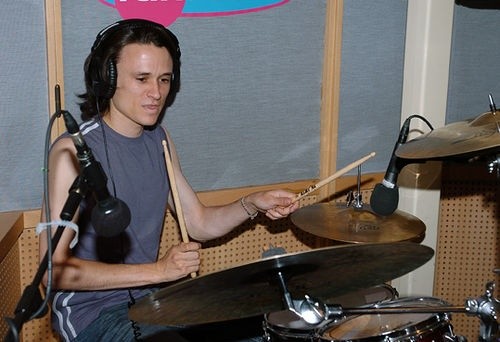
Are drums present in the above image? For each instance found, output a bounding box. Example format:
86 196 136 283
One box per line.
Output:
315 295 457 342
263 282 399 342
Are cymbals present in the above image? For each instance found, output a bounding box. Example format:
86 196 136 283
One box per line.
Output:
396 109 500 161
292 202 426 243
127 241 435 325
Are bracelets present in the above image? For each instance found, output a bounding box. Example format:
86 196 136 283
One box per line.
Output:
241 197 258 220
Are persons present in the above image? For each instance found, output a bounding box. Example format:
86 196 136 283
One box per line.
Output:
37 20 300 342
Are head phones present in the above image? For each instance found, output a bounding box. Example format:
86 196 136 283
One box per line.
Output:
84 18 183 103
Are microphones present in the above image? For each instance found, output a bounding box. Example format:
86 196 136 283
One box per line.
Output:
370 118 410 216
63 112 131 238
